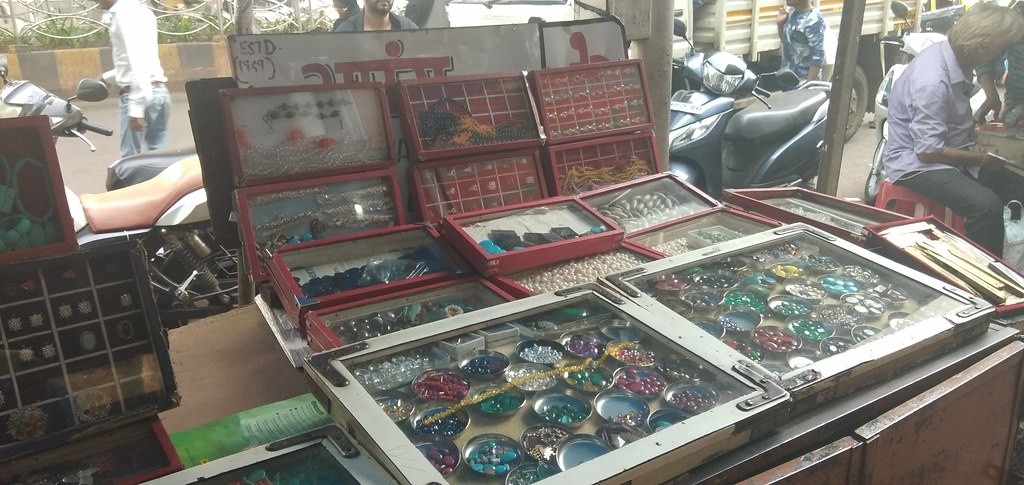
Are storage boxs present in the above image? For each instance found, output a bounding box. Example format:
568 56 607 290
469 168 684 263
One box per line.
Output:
138 422 401 485
215 57 1023 485
0 114 184 485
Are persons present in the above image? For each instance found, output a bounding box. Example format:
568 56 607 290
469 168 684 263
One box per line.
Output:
883 0 1024 260
332 0 419 31
96 0 172 158
776 0 825 83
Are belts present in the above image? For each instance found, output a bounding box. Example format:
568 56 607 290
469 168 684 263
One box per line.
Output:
119 82 164 96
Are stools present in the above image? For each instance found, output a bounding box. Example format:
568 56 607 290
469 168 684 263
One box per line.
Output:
875 179 967 232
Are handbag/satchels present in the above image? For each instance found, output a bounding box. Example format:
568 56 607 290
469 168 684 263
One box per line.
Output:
1002 200 1024 274
817 27 837 82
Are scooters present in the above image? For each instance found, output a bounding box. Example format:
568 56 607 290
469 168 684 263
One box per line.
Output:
0 54 239 328
864 0 989 209
668 8 834 201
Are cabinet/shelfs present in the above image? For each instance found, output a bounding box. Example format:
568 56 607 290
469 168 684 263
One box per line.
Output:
672 326 1023 484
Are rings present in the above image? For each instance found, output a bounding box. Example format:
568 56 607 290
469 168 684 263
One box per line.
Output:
422 157 536 214
0 263 134 362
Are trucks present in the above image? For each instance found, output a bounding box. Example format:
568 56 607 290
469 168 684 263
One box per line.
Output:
439 1 922 146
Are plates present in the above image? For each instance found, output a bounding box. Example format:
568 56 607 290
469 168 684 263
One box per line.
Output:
370 242 949 485
